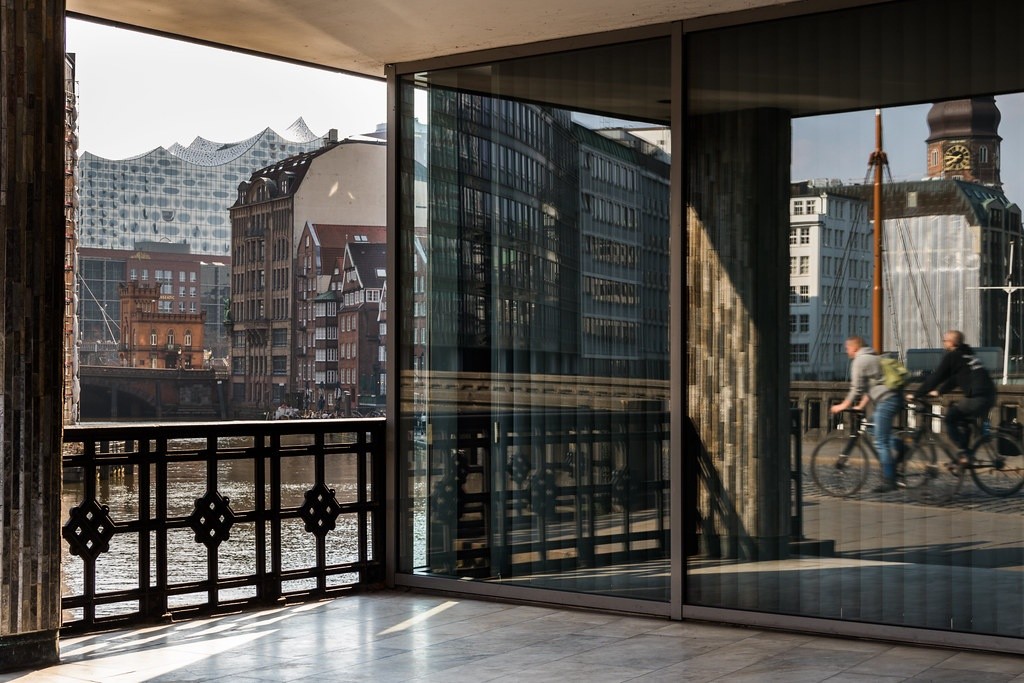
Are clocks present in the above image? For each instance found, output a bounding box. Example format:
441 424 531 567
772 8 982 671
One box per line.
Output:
943 144 971 171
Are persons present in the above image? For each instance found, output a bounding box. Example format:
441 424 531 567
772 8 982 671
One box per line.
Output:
911 329 1000 460
827 335 916 495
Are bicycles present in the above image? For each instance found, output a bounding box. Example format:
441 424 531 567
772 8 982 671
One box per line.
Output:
809 406 966 507
888 391 1024 496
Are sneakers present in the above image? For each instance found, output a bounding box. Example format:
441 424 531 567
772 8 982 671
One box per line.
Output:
947 448 969 466
894 445 909 462
872 479 898 492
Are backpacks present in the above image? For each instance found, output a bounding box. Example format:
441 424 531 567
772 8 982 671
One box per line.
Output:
880 356 910 390
